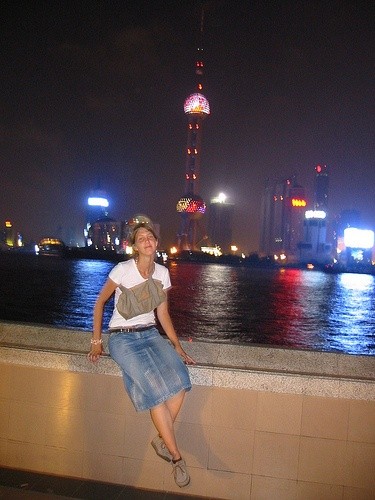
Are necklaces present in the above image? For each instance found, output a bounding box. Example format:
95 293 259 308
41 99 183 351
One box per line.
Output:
136 256 155 265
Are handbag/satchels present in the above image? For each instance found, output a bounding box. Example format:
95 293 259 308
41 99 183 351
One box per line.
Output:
116 278 166 320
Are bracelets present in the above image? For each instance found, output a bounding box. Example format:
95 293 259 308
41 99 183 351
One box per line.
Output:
91 338 103 345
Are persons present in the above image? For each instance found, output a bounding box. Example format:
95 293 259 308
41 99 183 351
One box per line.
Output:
89 223 195 487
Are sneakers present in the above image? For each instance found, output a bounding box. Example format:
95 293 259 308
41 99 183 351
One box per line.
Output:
150 436 171 462
172 458 190 488
